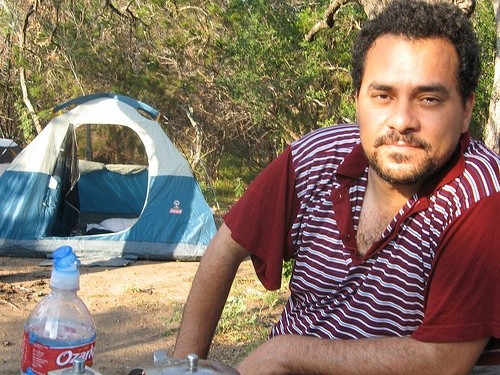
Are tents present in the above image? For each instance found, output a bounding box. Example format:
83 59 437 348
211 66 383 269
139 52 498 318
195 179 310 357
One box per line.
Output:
0 137 23 178
0 92 218 267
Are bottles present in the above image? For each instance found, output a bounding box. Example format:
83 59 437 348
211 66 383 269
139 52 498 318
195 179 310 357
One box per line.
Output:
19 246 97 375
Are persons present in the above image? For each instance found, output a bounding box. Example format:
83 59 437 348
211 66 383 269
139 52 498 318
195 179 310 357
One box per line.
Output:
168 0 500 375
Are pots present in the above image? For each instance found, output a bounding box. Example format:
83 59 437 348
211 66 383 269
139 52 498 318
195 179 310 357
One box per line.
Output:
144 349 240 375
